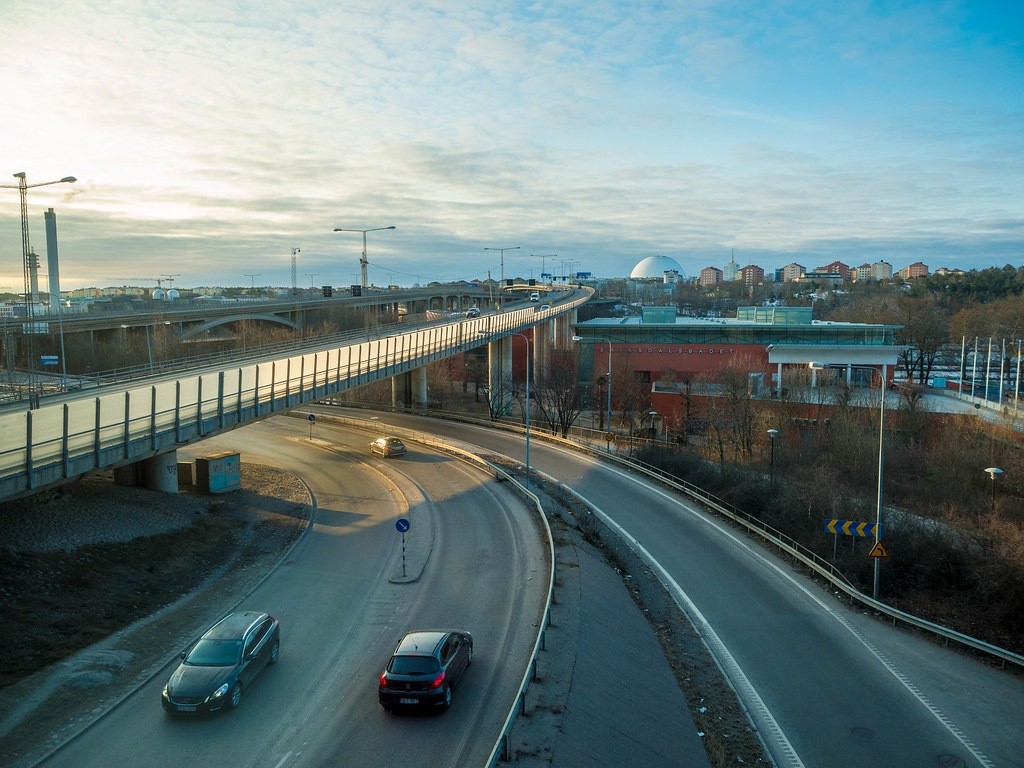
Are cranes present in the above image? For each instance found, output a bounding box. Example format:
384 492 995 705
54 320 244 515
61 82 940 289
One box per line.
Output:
106 277 174 288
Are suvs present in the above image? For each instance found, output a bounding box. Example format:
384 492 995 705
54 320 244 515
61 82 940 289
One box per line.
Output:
162 611 280 718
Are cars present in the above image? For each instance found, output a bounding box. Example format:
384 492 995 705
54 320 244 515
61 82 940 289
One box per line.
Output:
540 305 550 313
466 308 481 318
377 628 474 714
370 436 408 459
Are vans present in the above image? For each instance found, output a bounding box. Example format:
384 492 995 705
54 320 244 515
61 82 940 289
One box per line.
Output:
530 293 540 302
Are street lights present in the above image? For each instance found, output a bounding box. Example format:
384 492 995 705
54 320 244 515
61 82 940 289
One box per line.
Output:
0 171 77 314
333 226 397 290
349 273 362 285
386 273 397 285
245 274 262 287
984 468 1003 513
19 293 67 391
572 336 611 446
530 254 558 295
304 272 319 288
552 258 581 290
161 274 180 300
649 411 659 439
808 362 885 602
484 246 521 304
477 330 530 490
766 429 780 479
